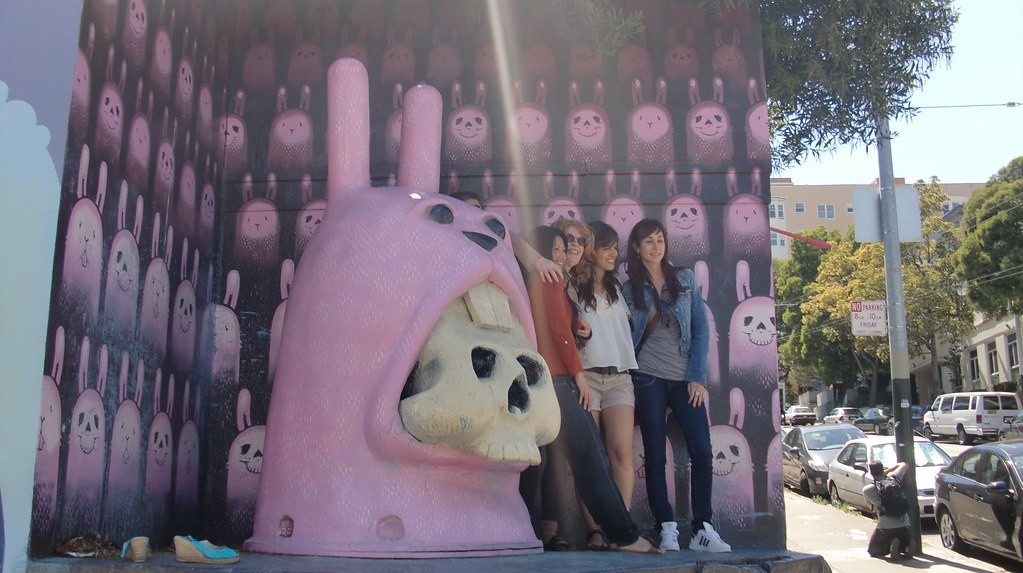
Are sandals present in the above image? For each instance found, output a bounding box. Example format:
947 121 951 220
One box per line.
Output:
544 533 568 551
587 531 608 550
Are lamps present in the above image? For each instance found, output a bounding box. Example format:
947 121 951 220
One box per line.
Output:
912 364 915 368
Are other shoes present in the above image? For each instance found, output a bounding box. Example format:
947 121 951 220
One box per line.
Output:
890 537 901 562
904 539 916 559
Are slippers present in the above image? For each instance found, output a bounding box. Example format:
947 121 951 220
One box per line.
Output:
616 537 665 554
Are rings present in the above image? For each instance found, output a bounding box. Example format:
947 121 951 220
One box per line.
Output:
695 391 699 395
540 272 543 274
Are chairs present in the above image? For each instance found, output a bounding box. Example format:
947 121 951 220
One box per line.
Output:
800 434 817 450
973 460 992 482
993 456 1021 488
826 431 846 446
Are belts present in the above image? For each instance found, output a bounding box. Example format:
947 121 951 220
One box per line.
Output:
588 366 624 375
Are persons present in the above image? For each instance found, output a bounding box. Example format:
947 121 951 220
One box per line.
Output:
863 460 916 561
459 191 666 553
621 218 730 553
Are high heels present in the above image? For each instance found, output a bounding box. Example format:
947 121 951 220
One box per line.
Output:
173 534 241 564
121 536 151 564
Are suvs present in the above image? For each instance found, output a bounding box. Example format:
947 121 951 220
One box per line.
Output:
924 390 1022 445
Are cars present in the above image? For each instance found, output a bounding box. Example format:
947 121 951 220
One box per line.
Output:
853 406 894 435
934 441 1023 561
782 425 875 499
781 404 818 426
822 406 863 426
887 405 931 435
827 436 968 523
998 410 1023 445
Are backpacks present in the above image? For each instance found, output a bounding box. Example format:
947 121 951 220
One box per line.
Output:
874 478 910 517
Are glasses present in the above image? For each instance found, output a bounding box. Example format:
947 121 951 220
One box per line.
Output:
566 233 587 246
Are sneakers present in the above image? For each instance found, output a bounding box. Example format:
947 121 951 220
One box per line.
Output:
658 520 680 551
688 522 731 553
